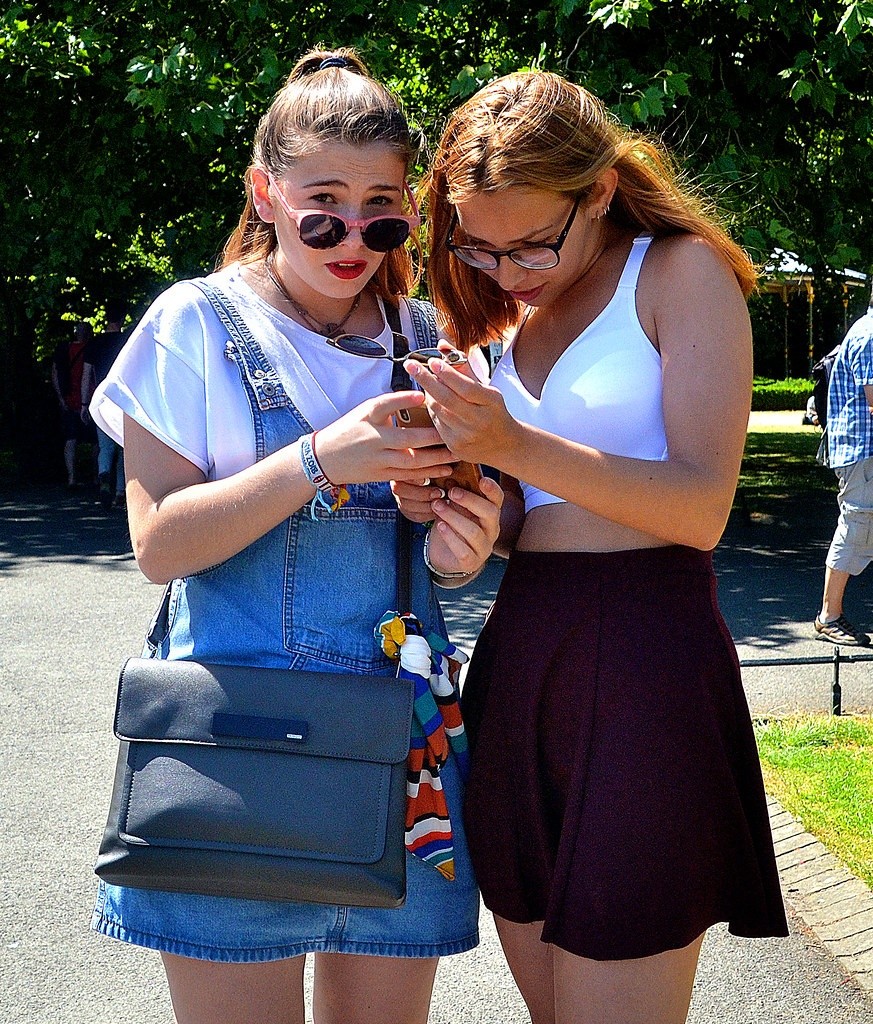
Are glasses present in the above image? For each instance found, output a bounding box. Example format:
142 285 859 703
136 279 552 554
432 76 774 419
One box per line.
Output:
325 334 468 368
267 171 421 255
444 190 581 271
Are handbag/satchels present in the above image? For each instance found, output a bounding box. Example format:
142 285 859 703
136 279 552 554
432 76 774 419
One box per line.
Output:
94 657 415 909
59 409 89 436
811 344 841 458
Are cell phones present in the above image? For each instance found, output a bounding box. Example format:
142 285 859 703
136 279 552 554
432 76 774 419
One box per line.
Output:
395 397 488 518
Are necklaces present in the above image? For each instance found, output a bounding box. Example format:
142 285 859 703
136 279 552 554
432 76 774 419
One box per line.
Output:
260 252 362 337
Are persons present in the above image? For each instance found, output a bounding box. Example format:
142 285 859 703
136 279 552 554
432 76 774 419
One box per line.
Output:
82 298 134 508
401 66 793 1024
813 283 873 647
50 321 96 491
82 45 508 1023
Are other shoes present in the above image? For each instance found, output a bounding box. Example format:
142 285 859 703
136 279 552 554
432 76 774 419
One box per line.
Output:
99 482 111 510
812 610 871 646
116 488 125 506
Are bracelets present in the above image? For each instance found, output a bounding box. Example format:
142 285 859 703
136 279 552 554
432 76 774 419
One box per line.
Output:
82 401 88 406
299 428 350 522
422 527 476 579
